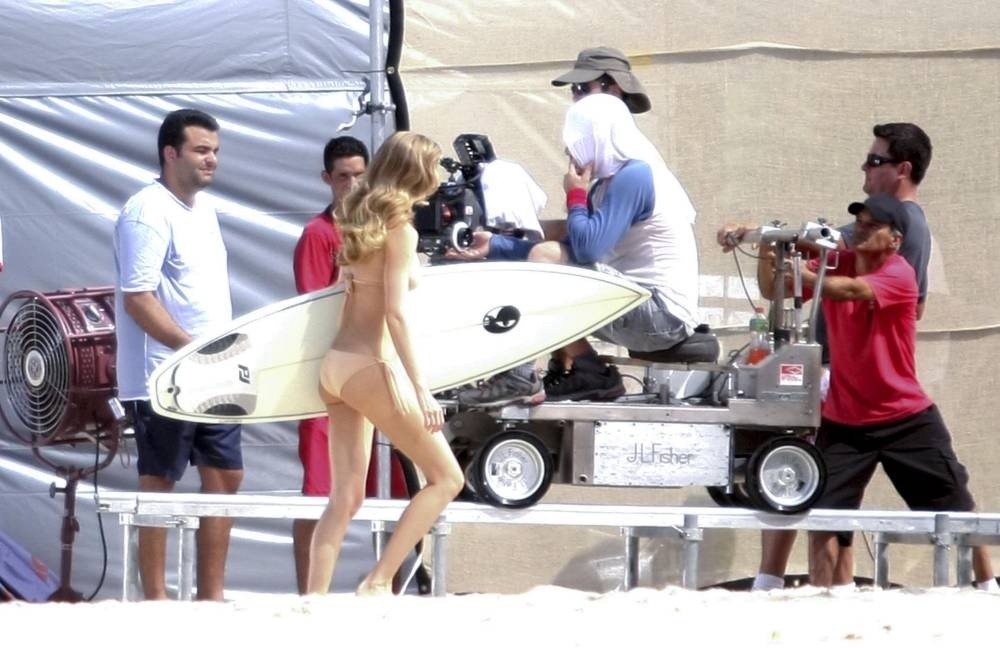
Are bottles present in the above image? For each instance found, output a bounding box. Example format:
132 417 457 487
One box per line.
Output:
749 305 772 366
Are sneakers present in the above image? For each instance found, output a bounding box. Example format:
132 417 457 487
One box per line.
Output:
541 349 626 403
457 369 545 408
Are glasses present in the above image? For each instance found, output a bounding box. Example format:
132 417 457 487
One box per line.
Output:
570 81 607 95
867 153 900 168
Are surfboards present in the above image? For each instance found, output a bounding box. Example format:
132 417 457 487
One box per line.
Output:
146 263 651 423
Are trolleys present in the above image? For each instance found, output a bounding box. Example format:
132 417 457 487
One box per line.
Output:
430 224 845 515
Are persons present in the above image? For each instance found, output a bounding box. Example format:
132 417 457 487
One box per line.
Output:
113 108 243 602
291 135 410 595
304 131 466 597
456 45 998 591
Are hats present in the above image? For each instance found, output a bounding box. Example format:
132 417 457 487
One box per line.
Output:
552 46 651 113
848 191 910 237
